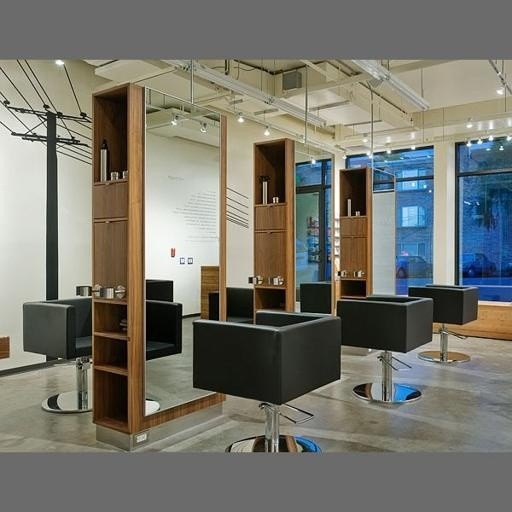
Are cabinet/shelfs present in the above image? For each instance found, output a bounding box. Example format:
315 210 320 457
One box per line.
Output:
92 83 142 435
338 167 371 303
252 140 294 318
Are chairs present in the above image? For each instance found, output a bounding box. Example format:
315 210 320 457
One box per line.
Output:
22 297 93 413
191 308 342 454
408 284 478 363
146 299 182 361
337 294 433 404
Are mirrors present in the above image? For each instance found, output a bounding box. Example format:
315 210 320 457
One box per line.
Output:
291 141 333 316
145 89 228 430
373 170 396 297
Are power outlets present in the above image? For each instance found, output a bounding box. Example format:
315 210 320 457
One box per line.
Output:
180 258 185 264
188 258 193 264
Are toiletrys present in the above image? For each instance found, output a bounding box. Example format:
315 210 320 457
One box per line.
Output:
110 172 119 180
123 171 129 179
272 197 278 204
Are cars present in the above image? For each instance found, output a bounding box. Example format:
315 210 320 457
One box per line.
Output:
395 255 432 279
462 252 497 278
